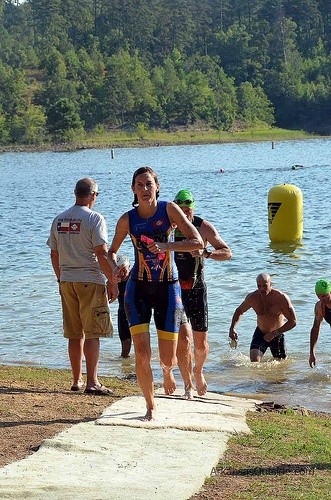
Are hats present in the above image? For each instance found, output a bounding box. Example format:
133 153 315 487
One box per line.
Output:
174 189 194 207
315 279 331 294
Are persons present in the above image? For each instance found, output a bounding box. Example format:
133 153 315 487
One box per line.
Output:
46 178 120 394
106 254 131 358
107 167 205 421
309 279 331 368
229 272 297 362
172 189 232 399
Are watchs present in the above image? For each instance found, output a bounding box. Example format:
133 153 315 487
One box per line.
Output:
204 248 212 258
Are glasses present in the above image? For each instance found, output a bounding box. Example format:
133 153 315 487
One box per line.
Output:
94 192 99 196
173 200 194 204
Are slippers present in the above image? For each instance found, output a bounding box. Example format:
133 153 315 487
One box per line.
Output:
71 381 86 391
84 383 113 395
